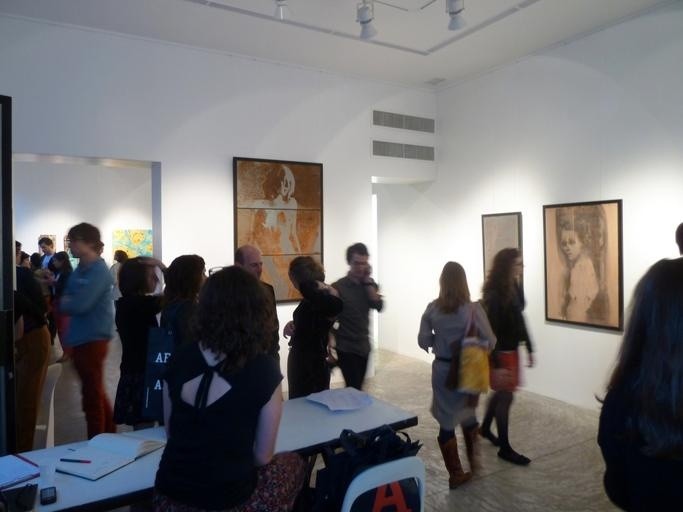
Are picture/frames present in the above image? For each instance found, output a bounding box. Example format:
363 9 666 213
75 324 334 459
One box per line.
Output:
479 210 524 305
539 198 626 335
230 154 326 304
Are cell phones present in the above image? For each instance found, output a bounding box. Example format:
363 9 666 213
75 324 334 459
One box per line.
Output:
40 487 56 504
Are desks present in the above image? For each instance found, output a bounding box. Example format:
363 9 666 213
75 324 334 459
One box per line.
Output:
0 384 419 511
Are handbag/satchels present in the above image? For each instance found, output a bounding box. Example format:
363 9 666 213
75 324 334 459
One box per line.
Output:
140 366 162 421
146 327 176 377
315 424 424 511
445 335 490 393
325 344 338 368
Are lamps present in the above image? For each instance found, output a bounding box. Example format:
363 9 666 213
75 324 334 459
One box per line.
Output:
354 0 378 40
444 0 465 31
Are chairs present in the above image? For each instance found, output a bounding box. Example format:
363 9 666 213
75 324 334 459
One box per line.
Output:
336 455 429 512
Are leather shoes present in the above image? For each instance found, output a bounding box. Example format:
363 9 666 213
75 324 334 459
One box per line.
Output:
498 448 531 465
478 427 501 446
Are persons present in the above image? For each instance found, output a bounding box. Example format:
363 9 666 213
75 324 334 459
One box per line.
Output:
417 262 499 489
246 166 303 299
150 264 308 511
16 223 114 451
283 256 343 400
552 213 602 324
330 242 383 389
594 257 682 511
110 244 277 432
475 248 536 470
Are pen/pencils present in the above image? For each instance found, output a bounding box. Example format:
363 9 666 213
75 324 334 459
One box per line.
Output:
14 453 38 467
60 458 91 463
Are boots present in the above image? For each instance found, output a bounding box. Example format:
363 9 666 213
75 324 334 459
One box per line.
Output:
464 423 487 473
437 435 472 490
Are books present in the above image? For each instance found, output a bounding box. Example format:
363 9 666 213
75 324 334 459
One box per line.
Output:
53 431 164 483
0 452 43 489
